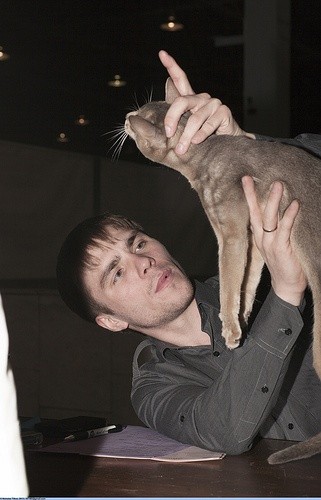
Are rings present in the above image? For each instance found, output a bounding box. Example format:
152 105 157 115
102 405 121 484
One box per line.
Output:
262 226 277 233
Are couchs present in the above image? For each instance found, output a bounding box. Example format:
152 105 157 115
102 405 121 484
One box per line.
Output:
1 140 219 428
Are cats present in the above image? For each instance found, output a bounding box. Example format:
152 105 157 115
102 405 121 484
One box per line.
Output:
107 77 320 464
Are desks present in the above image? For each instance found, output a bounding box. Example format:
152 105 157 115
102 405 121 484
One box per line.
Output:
18 415 321 497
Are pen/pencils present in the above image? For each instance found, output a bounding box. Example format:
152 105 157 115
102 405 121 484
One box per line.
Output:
64 424 123 442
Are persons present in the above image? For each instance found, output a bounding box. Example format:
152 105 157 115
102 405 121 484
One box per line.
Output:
54 175 321 457
